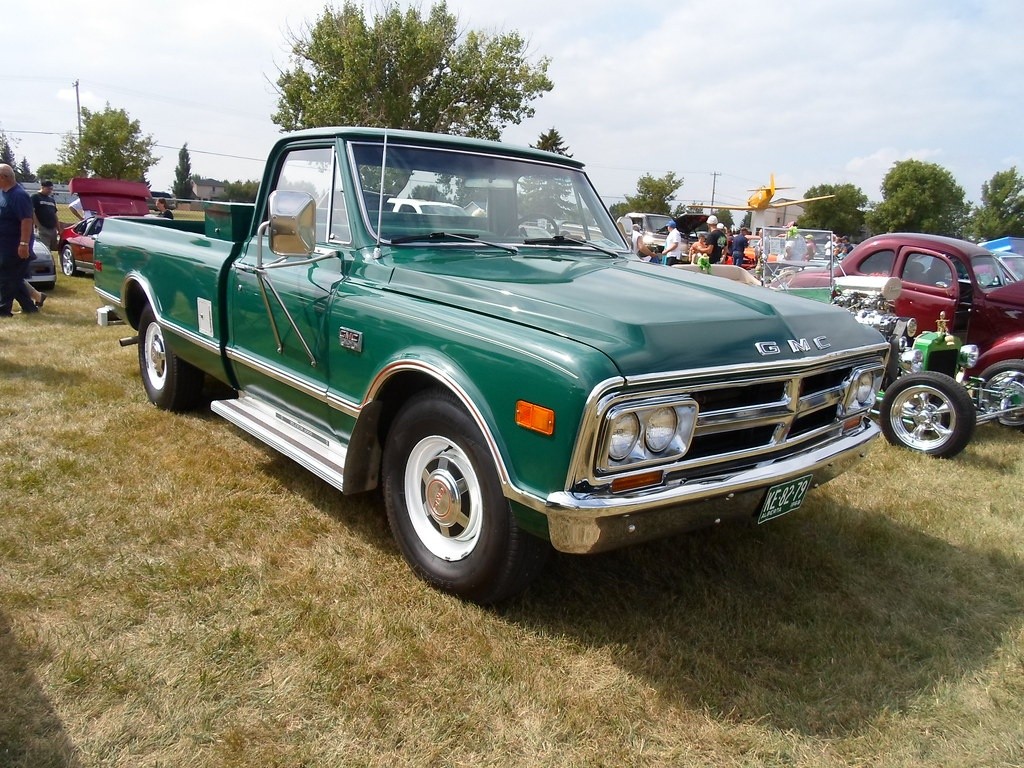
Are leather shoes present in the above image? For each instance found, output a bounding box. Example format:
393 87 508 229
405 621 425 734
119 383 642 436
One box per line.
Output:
34 292 46 307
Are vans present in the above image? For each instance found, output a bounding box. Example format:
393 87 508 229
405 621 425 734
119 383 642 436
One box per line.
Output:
146 191 177 211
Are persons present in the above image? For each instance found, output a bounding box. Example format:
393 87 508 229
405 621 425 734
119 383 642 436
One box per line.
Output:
619 214 853 267
31 181 64 255
24 278 47 308
0 163 39 318
68 197 102 230
155 198 174 220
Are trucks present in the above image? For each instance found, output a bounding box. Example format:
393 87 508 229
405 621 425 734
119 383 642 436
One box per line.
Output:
558 213 711 264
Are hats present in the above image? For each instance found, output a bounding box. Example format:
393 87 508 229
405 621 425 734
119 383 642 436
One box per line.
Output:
633 224 640 232
665 221 676 227
785 221 797 228
41 180 53 187
805 234 814 239
707 215 718 224
840 236 849 240
717 223 725 229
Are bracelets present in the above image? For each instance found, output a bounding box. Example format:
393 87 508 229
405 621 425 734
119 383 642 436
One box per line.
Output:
19 241 29 246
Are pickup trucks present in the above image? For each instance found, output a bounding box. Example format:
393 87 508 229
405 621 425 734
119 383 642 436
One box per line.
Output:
93 127 891 606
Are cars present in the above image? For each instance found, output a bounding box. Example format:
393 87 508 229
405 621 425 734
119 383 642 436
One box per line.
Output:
386 198 469 216
731 235 777 263
58 177 172 276
671 226 1024 460
910 251 1024 288
689 232 710 242
767 233 1023 382
518 225 553 238
24 237 57 291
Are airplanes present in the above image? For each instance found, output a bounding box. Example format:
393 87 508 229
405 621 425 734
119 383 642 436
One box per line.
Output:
687 170 835 213
